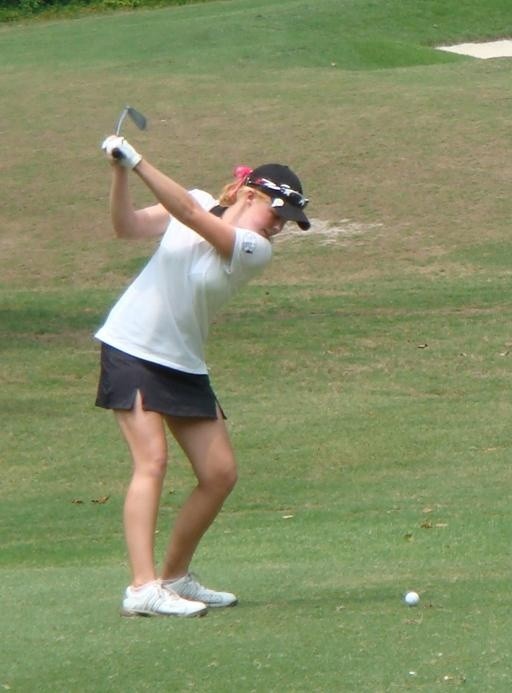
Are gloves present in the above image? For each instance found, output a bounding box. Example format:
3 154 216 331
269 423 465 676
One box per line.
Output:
101 137 143 170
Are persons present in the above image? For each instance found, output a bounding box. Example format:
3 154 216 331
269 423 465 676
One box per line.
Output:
93 130 314 621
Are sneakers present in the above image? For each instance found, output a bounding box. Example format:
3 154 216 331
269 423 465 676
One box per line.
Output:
155 573 240 609
120 578 207 621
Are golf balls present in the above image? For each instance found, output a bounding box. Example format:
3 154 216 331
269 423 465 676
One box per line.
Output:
405 592 419 605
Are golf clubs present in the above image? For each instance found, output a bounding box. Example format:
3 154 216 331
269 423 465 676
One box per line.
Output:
111 104 146 158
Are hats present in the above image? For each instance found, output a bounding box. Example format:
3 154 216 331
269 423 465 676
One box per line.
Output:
242 162 312 232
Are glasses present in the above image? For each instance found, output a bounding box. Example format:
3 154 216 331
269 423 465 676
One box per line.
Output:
263 179 310 210
